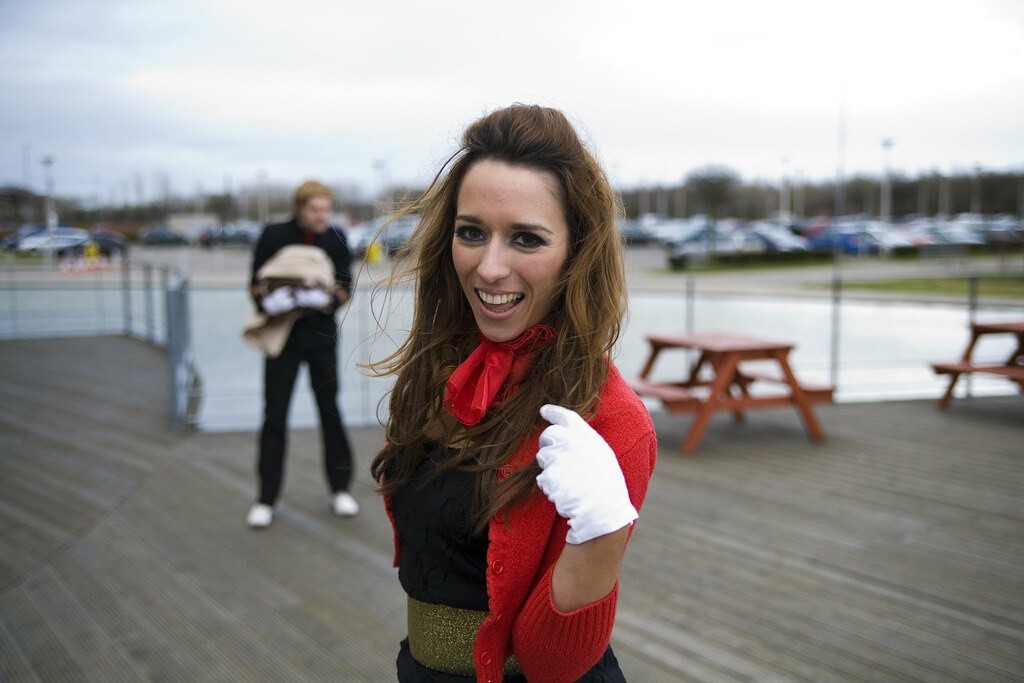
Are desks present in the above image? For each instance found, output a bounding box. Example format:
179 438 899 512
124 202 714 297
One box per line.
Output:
636 333 827 456
937 322 1024 412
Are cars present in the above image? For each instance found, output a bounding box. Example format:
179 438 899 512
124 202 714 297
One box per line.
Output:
2 213 1022 263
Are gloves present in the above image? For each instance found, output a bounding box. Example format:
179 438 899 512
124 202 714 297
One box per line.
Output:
264 286 294 314
532 405 641 547
296 287 331 310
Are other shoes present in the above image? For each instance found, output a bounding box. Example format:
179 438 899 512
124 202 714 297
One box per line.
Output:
330 490 360 517
247 501 272 527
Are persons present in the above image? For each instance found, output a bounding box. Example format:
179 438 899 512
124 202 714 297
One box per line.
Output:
246 180 360 526
357 101 658 682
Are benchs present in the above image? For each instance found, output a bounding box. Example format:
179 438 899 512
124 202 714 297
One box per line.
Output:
628 380 701 414
933 361 1024 384
745 371 835 406
